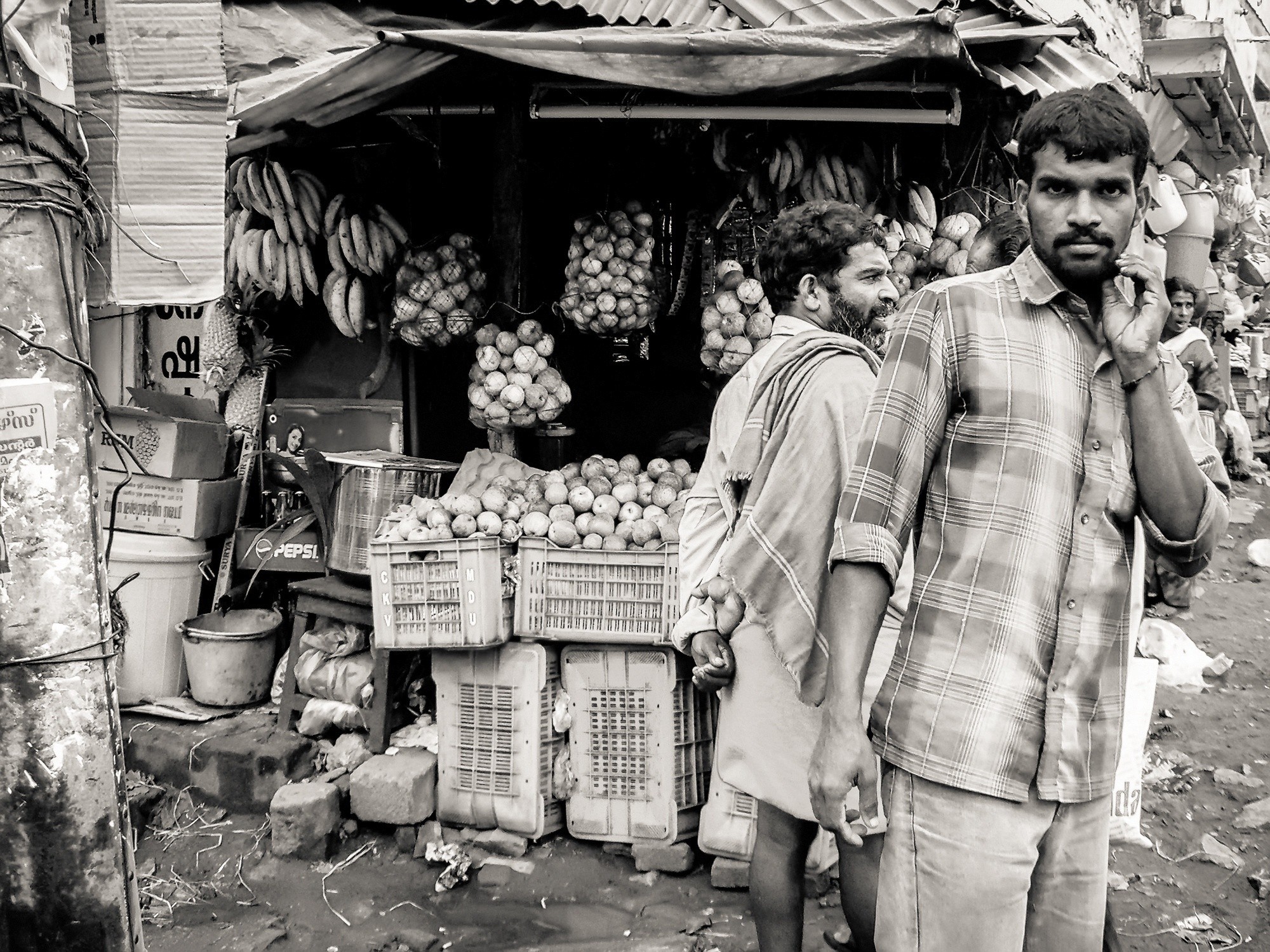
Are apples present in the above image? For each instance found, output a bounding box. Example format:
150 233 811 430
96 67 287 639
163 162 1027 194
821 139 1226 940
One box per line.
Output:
393 231 489 347
878 211 986 361
560 202 660 339
468 319 571 433
385 455 699 561
700 259 776 377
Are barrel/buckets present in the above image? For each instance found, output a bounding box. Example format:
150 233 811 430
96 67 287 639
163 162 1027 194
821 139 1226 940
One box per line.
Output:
176 603 287 707
329 458 443 579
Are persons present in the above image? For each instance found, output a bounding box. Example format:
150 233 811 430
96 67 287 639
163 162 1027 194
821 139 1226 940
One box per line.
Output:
265 422 307 456
803 81 1234 950
668 195 914 952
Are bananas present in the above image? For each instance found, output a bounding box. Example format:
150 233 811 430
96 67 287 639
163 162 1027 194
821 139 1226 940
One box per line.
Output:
712 122 937 254
224 156 410 338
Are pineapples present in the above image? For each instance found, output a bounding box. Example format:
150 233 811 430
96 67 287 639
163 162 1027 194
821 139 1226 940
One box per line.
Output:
200 276 291 437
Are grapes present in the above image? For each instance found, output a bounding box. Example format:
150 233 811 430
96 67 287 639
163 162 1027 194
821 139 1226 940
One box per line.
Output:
134 418 160 471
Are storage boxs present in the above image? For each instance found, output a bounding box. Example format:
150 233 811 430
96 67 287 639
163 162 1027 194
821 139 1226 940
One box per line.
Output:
1228 325 1269 441
91 381 839 876
